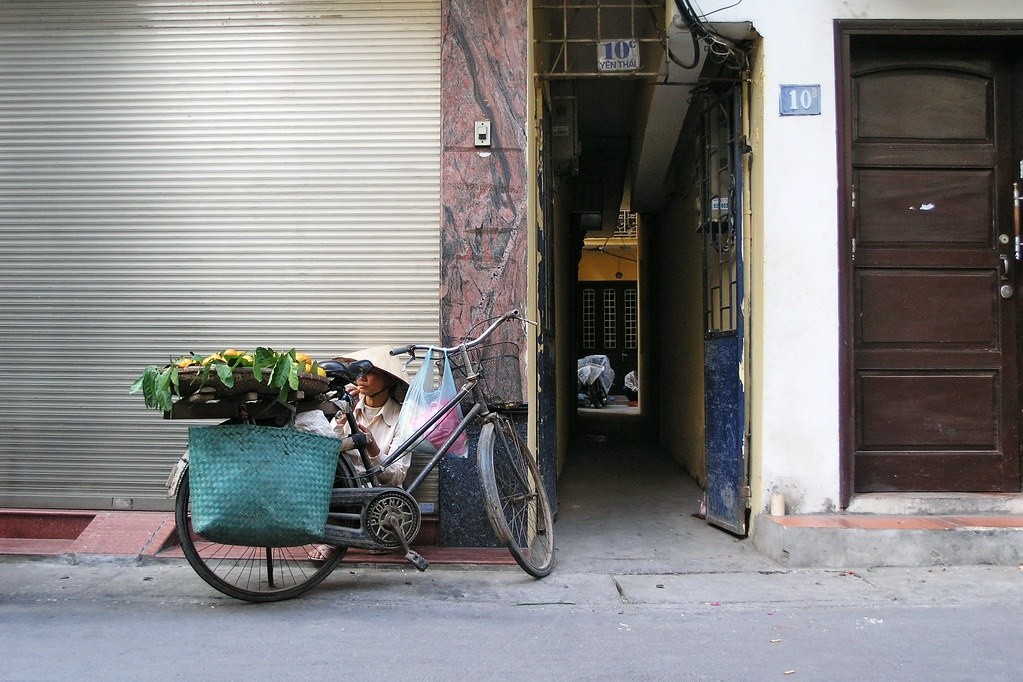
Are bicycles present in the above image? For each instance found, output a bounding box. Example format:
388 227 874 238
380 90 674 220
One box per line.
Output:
160 308 556 605
579 355 612 407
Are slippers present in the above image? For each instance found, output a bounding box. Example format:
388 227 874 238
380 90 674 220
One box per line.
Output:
307 544 339 562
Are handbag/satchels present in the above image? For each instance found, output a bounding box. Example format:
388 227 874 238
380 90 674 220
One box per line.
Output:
398 346 469 458
186 399 343 549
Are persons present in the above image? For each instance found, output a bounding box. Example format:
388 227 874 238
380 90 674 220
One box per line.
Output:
308 355 414 562
622 363 638 401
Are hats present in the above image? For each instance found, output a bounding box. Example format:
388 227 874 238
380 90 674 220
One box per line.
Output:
332 346 413 404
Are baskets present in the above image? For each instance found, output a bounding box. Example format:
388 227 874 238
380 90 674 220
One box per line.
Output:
439 340 523 417
155 365 332 397
